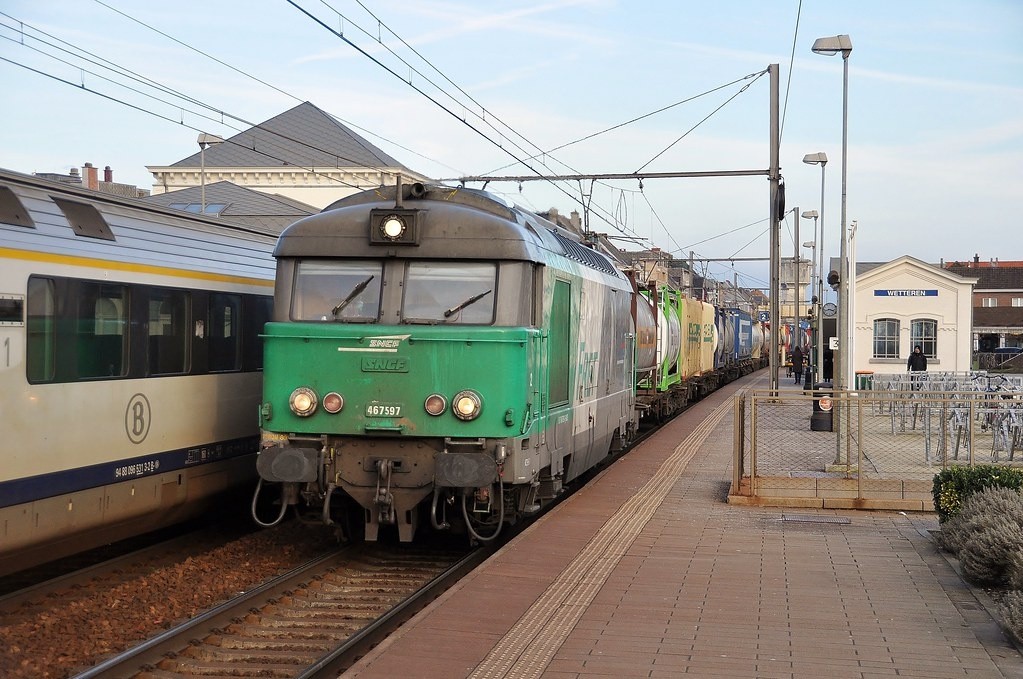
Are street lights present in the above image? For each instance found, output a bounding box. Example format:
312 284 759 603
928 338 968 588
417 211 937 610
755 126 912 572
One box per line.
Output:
801 210 818 318
803 240 814 299
801 150 825 388
811 32 859 474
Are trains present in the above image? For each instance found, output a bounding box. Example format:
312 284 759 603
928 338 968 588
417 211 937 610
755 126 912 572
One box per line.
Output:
248 179 788 550
2 167 278 573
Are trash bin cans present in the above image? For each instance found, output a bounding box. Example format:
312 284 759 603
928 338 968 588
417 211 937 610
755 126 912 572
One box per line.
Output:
810 383 833 432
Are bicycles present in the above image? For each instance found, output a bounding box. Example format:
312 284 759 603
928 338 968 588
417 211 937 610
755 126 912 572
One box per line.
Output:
971 370 1023 457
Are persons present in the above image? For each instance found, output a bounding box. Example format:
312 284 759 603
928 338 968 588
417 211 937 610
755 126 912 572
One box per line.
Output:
792 346 803 384
906 345 927 398
